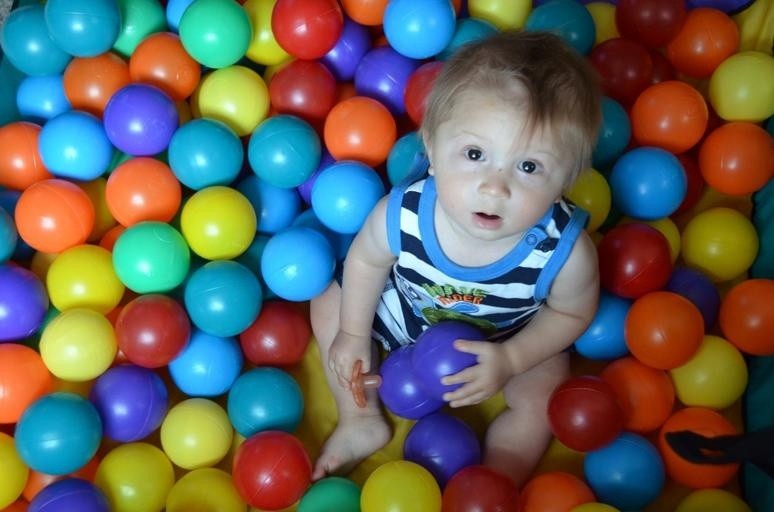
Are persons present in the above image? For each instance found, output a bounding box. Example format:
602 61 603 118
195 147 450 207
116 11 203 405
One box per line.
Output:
307 28 601 489
